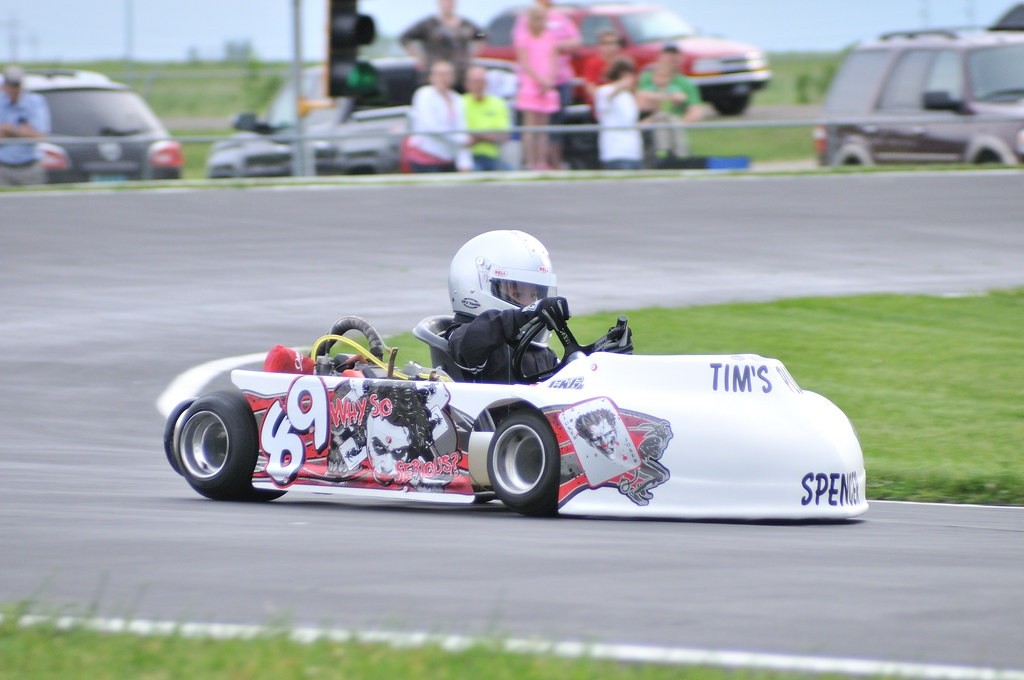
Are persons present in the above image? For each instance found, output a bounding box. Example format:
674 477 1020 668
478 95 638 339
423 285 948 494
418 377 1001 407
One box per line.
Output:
394 0 706 173
446 230 633 384
0 65 51 185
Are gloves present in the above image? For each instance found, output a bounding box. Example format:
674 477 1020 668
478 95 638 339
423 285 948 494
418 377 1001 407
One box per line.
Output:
608 326 632 353
521 296 572 332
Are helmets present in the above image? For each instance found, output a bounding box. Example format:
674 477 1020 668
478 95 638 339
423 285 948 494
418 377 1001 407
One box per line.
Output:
447 228 557 349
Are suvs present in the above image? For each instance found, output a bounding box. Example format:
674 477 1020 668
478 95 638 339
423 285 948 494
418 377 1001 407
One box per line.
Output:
814 29 1024 174
26 68 185 183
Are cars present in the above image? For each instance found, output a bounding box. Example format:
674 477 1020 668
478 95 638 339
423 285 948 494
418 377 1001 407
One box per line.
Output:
209 59 595 177
468 3 772 126
